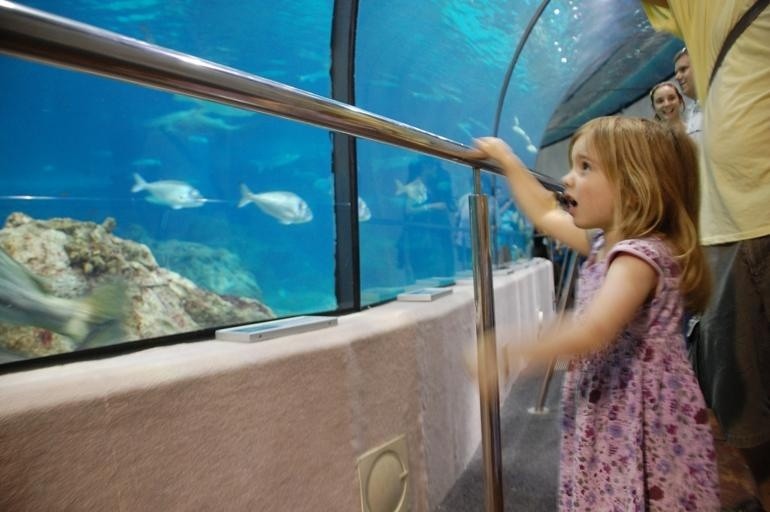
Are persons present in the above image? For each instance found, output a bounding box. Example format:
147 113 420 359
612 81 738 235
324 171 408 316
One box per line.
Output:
644 0 770 511
672 47 735 437
472 114 724 512
649 81 687 135
